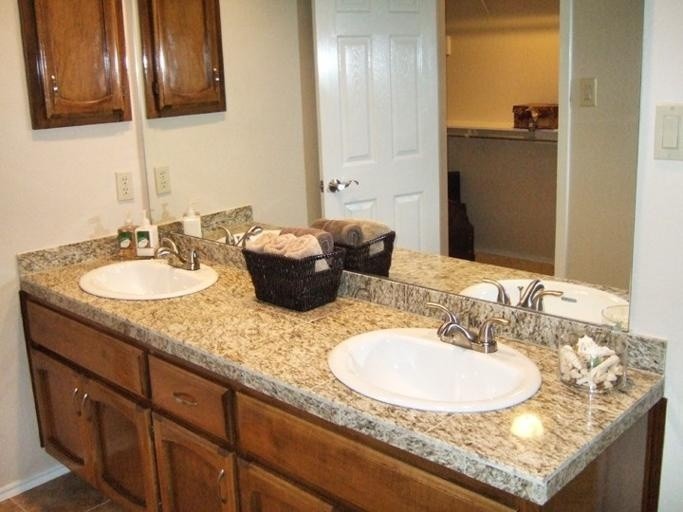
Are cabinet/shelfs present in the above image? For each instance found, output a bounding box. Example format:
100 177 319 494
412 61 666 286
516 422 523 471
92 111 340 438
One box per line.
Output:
22 298 161 512
19 0 133 131
138 0 227 120
147 356 235 511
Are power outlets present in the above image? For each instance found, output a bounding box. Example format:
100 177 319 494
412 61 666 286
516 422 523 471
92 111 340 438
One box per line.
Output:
118 172 134 203
154 166 172 196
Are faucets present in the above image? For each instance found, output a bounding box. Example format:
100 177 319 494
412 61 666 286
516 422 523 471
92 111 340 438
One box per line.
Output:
437 320 478 348
156 246 186 268
234 225 263 247
515 278 544 307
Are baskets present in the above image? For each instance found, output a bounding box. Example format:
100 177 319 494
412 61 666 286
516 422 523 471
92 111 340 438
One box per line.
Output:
334 230 395 278
241 246 346 312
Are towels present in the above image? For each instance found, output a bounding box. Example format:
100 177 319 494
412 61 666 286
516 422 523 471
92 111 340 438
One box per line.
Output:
251 226 334 271
310 218 392 258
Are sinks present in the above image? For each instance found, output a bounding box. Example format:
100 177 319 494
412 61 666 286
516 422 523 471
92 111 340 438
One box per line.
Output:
327 327 544 413
459 276 629 330
79 259 221 301
216 229 280 247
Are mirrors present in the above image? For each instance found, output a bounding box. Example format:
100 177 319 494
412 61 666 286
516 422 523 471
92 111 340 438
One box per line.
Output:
125 0 648 333
